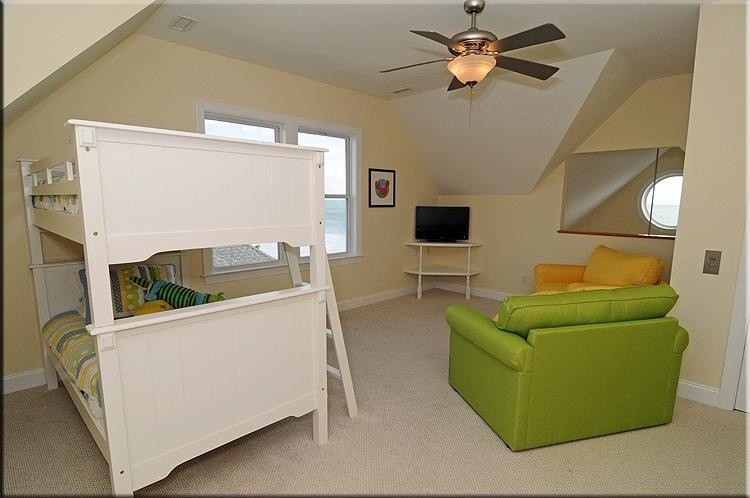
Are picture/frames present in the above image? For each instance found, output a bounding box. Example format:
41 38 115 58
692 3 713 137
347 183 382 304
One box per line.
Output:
368 168 396 208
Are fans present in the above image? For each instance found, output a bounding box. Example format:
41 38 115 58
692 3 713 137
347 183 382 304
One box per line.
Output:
379 1 566 129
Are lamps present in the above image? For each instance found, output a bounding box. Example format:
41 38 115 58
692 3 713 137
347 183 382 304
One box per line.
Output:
446 49 497 89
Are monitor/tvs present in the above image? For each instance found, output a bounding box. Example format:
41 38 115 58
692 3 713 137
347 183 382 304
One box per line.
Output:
415 206 470 242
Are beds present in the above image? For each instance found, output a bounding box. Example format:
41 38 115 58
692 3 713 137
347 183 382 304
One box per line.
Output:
15 119 360 498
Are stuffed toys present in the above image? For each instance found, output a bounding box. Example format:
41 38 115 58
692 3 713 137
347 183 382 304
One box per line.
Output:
134 300 171 315
130 273 225 309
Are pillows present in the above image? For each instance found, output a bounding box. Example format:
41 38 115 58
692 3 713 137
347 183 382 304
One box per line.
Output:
72 263 227 324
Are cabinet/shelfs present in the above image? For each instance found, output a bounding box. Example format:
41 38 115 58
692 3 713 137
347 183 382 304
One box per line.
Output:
403 242 481 300
